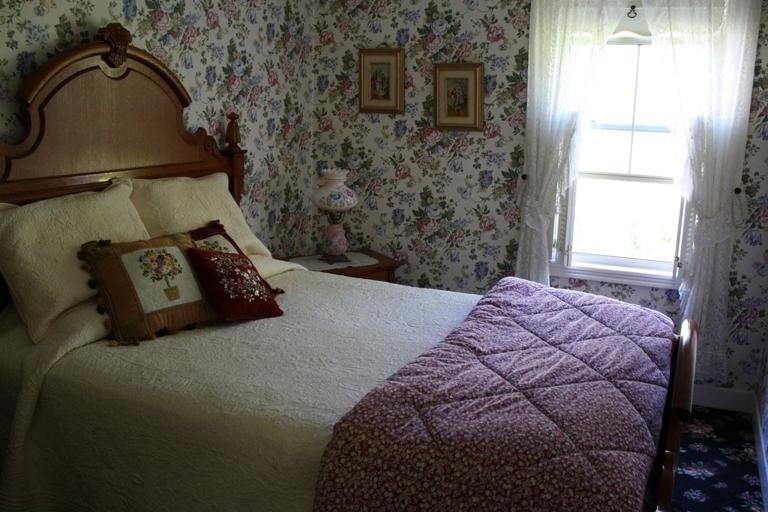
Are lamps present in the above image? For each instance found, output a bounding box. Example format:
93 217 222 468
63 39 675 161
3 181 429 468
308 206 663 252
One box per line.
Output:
311 167 359 264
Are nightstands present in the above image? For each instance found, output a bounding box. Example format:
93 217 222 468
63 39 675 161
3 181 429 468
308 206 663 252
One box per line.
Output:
286 247 394 286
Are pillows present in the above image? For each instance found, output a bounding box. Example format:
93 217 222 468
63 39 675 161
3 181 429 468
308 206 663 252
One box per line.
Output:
108 172 272 260
184 246 284 325
76 231 222 347
186 218 286 299
0 176 154 345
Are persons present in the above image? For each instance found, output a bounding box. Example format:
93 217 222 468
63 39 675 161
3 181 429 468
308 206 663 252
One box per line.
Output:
370 71 389 93
446 86 467 107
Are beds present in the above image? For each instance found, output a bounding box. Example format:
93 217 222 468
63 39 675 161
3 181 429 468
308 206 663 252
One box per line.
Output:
0 23 698 511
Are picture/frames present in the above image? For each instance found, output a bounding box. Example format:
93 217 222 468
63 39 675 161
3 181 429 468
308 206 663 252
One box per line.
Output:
432 61 486 131
356 48 406 114
